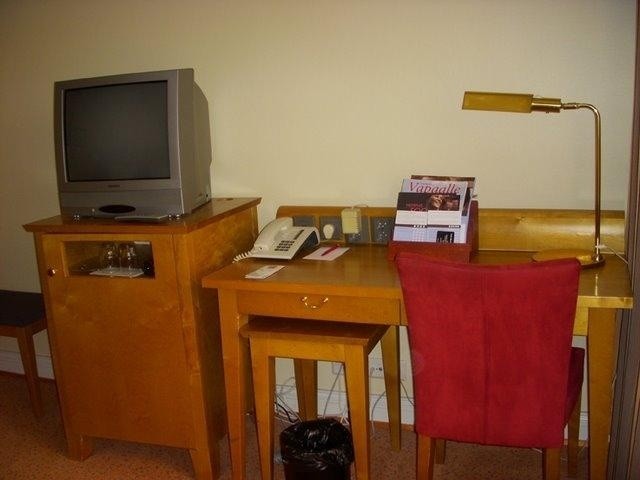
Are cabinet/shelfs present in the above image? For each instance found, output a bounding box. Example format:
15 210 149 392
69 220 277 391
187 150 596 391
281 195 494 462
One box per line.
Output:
24 197 263 476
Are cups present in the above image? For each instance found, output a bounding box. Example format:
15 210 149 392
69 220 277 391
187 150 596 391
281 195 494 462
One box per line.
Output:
98 242 139 274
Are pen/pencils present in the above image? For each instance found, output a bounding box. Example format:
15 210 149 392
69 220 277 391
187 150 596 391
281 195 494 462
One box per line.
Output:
321 244 340 257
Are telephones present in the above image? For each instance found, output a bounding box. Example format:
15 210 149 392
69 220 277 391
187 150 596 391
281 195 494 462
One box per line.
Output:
247 217 321 260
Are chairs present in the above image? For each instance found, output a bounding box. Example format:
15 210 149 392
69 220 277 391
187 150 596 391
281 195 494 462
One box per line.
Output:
391 252 586 478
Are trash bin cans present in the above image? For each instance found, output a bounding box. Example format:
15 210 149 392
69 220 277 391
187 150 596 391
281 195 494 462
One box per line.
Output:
280 419 354 480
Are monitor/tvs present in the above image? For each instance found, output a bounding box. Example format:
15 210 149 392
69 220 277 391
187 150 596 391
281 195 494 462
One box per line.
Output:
54 68 212 222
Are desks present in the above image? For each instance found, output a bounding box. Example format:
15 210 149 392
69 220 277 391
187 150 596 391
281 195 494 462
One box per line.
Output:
201 204 635 474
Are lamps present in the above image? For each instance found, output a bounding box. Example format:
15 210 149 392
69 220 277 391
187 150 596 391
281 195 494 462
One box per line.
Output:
461 90 606 269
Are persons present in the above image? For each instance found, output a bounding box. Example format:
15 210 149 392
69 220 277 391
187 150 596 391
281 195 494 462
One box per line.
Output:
425 194 458 211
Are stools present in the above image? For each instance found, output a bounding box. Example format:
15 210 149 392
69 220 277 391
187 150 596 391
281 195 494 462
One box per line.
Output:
239 317 403 479
0 290 48 419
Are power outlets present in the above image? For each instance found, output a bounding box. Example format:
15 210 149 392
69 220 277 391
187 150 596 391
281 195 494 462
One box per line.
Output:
365 358 404 380
332 361 345 374
